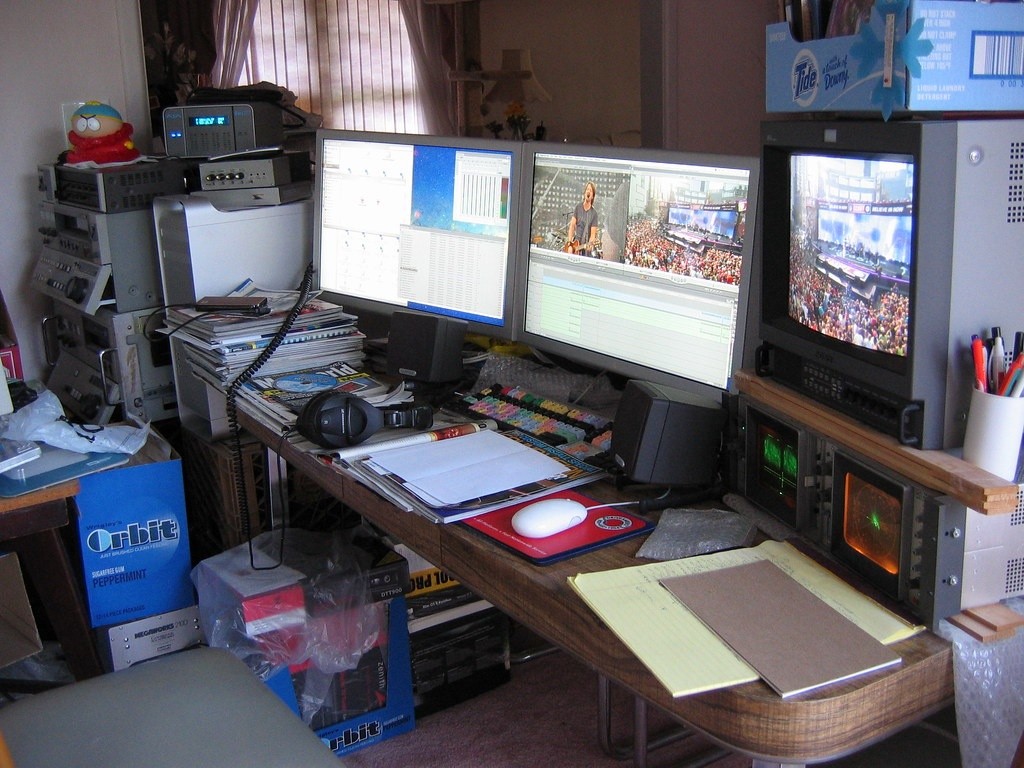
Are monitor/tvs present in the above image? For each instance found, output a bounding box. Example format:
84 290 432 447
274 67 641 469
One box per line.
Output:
511 139 762 419
312 128 529 387
752 119 1024 449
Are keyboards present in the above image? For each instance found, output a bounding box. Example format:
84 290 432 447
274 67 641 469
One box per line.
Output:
441 384 624 449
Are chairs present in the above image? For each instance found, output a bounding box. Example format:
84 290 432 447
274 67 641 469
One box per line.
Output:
0 646 353 768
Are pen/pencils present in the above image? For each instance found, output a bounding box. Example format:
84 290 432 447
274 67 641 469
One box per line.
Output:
970 327 1024 398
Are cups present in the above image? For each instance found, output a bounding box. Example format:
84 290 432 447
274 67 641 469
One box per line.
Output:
962 383 1024 480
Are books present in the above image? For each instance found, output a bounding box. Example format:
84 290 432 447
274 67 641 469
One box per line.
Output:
658 558 903 697
290 405 611 525
160 282 413 439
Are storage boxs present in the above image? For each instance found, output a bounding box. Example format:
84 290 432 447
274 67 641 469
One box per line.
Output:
765 0 1024 122
61 418 512 761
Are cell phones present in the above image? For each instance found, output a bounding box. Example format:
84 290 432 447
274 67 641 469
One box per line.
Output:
195 297 266 313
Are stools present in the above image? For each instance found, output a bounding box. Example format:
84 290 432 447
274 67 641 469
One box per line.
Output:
0 496 105 713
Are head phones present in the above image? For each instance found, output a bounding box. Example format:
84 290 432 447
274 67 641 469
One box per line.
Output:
295 391 435 450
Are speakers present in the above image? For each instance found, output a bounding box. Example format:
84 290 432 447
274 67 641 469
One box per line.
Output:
610 379 729 489
386 310 469 405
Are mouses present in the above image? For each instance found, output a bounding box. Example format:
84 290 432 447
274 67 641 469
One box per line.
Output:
511 499 587 538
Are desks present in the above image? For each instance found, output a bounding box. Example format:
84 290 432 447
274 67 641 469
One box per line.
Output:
228 301 958 768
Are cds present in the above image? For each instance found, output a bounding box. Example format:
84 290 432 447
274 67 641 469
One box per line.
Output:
274 374 337 393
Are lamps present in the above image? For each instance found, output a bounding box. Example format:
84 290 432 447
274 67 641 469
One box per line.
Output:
483 47 551 142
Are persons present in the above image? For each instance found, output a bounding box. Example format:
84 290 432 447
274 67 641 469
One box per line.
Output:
789 217 909 355
628 194 746 287
600 180 629 261
566 181 599 257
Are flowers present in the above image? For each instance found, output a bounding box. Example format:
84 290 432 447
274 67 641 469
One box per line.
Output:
144 20 201 109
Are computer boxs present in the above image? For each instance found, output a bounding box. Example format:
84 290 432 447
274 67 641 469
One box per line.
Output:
152 194 314 443
